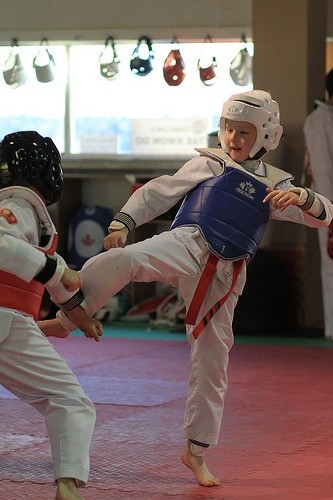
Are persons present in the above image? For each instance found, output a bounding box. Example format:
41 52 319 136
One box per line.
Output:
38 91 333 487
304 68 333 339
0 132 103 500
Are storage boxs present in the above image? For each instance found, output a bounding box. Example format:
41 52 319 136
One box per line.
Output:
208 131 286 168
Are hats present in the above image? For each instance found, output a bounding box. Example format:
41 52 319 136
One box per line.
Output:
33 50 57 84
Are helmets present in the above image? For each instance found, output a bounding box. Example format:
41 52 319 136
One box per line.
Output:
229 49 256 87
2 54 22 87
214 90 284 161
99 47 119 80
129 46 154 75
197 56 223 86
0 130 66 205
163 48 186 87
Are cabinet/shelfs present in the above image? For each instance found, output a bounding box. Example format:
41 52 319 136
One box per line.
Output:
53 174 274 307
